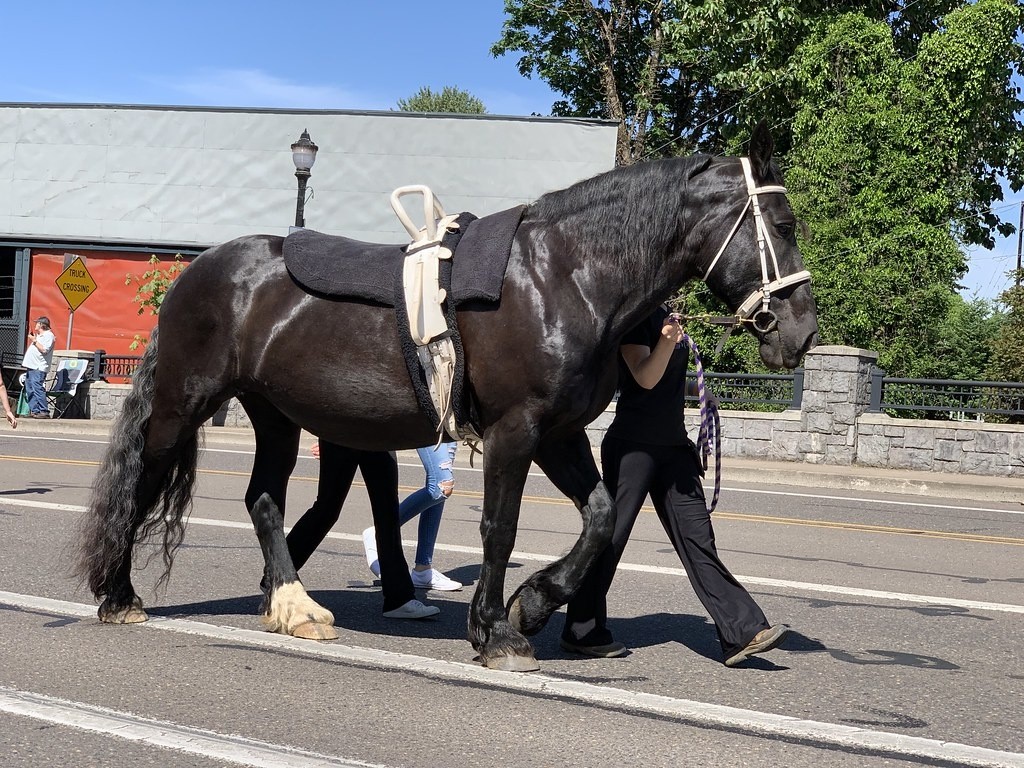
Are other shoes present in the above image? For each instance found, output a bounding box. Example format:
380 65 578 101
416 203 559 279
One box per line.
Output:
725 624 789 665
23 412 40 417
33 411 50 419
560 641 626 658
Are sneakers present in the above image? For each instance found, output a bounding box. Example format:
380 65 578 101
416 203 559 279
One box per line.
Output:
363 526 379 578
410 567 462 591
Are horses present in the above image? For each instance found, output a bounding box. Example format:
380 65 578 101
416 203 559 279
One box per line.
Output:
59 118 821 672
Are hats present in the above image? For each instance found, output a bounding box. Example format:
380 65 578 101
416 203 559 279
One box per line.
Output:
34 317 51 328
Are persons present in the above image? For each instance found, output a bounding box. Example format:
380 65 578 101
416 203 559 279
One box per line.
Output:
260 438 442 620
558 295 790 667
21 316 56 419
361 442 463 591
0 368 17 429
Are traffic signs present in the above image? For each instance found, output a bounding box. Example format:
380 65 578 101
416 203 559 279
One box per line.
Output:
55 257 96 312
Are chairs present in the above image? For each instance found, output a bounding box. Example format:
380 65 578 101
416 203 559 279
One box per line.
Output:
43 358 90 420
